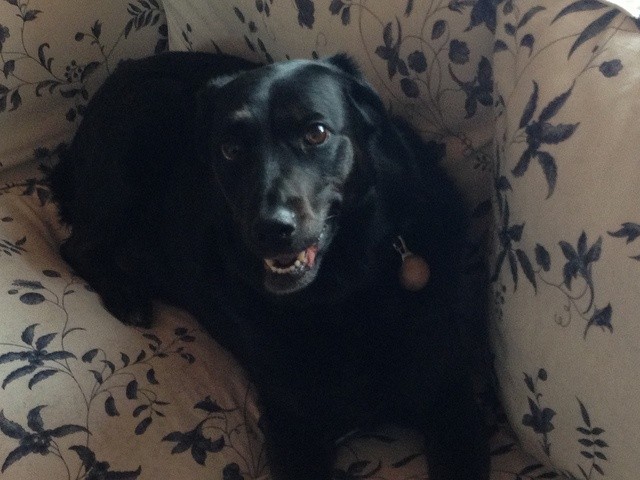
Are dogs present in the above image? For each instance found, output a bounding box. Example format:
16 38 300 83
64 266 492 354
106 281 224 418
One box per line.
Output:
45 48 498 480
